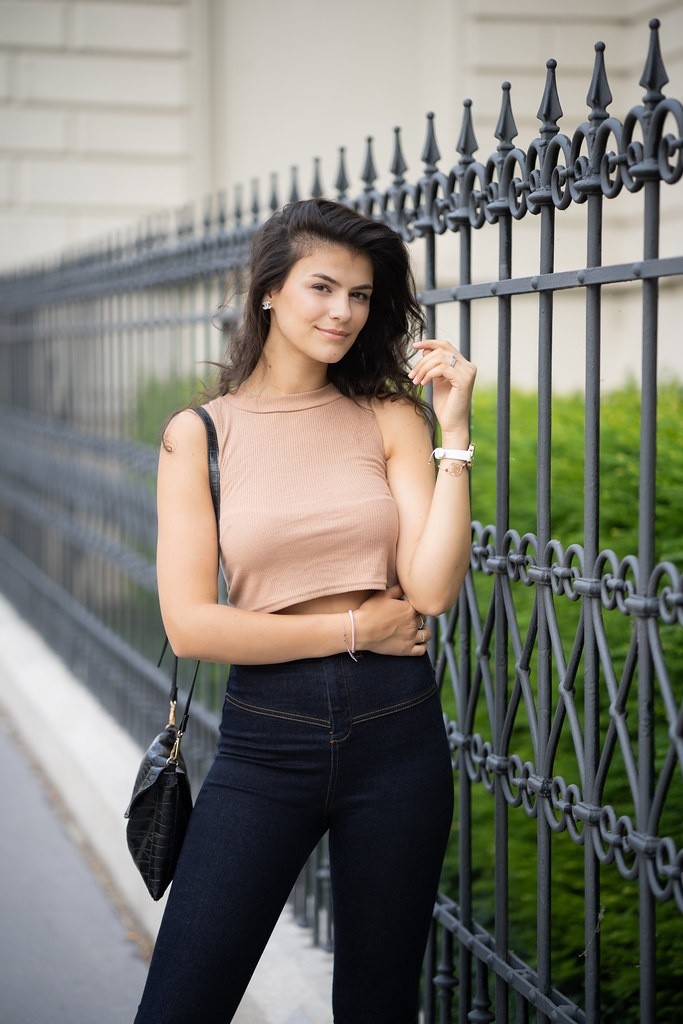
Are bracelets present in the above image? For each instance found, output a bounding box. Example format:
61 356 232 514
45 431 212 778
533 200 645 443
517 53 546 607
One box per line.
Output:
437 462 467 477
341 607 359 664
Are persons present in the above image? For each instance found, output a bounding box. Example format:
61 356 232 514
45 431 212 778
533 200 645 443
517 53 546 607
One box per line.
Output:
134 198 478 1024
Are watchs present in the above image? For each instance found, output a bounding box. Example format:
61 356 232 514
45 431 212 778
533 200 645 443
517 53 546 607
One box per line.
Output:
428 442 475 470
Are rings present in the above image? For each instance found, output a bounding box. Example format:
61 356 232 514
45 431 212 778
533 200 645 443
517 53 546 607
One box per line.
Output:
421 631 426 642
451 356 457 367
418 615 424 629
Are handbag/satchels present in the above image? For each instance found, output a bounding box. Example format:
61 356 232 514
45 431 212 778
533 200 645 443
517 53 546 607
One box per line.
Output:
123 731 195 902
